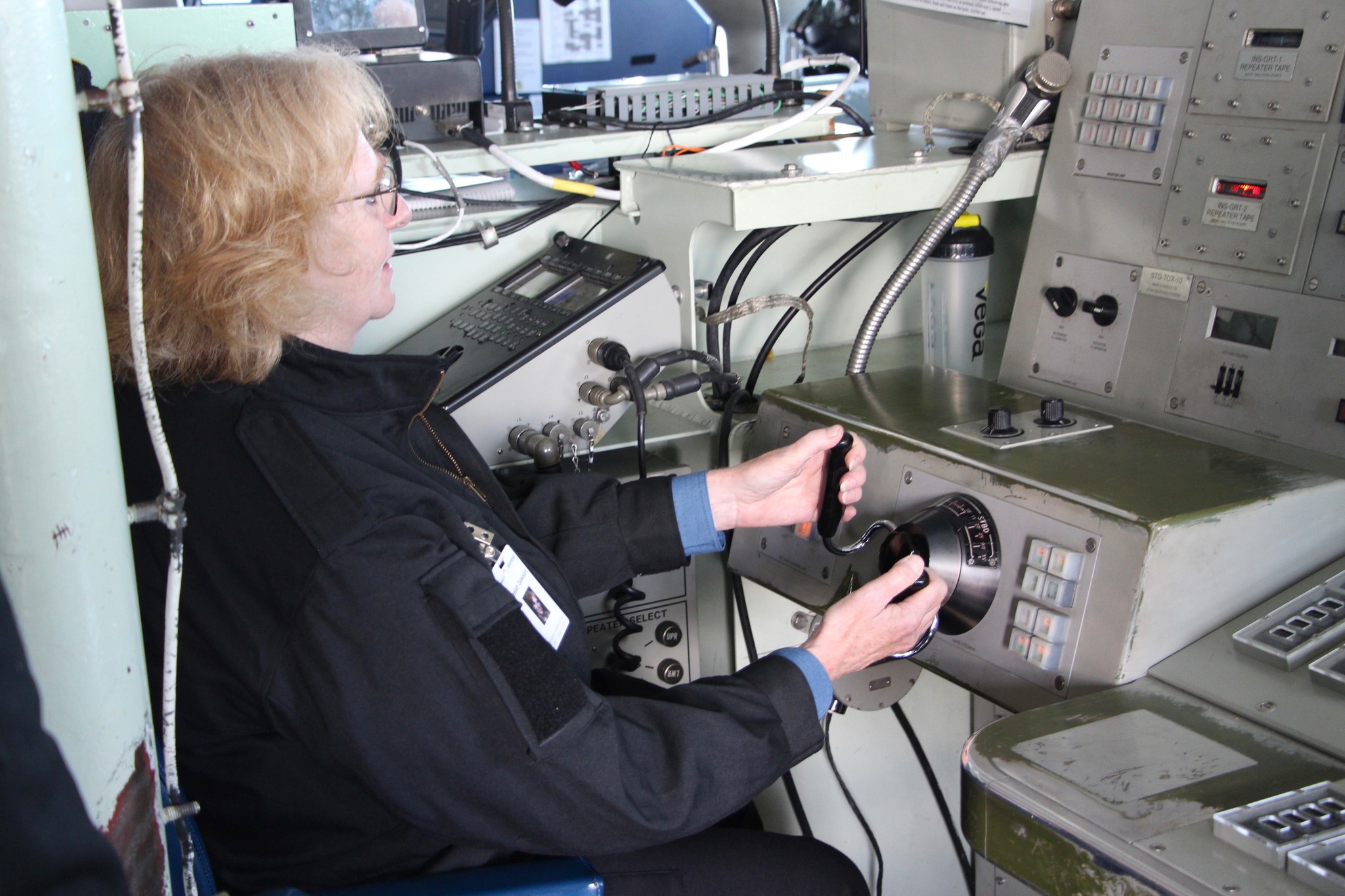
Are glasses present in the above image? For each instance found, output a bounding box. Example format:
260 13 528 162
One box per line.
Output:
309 161 400 218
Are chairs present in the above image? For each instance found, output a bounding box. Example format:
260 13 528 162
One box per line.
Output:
156 738 607 896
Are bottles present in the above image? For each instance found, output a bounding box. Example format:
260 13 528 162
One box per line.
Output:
920 215 994 379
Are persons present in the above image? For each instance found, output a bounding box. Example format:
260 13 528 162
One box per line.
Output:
91 43 950 896
